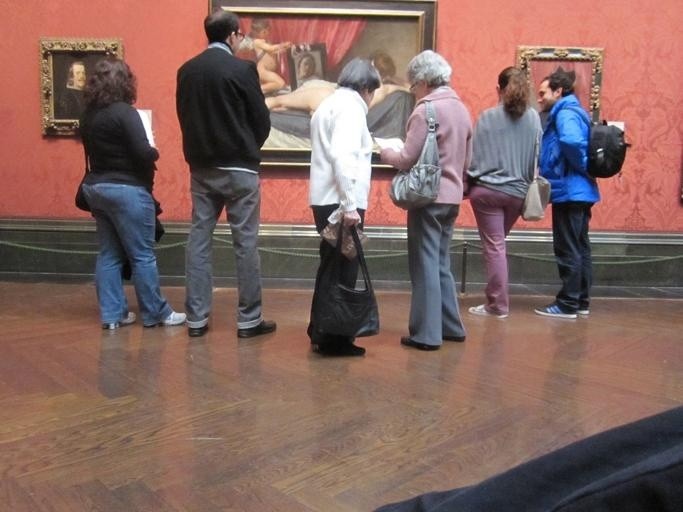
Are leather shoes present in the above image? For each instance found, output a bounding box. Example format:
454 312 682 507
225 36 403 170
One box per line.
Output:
401 335 465 350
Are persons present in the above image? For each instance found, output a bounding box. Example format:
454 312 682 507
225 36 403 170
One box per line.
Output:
54 62 86 118
533 73 600 319
266 52 405 110
308 56 380 357
371 48 471 350
80 60 185 329
468 67 541 319
176 11 277 339
249 20 293 98
295 53 321 84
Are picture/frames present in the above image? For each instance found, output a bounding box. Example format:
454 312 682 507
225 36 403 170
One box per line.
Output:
40 40 123 136
286 42 328 92
208 0 437 169
516 46 603 122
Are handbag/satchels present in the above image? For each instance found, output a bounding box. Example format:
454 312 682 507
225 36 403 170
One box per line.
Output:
75 170 92 212
316 281 379 336
523 176 550 221
389 101 442 208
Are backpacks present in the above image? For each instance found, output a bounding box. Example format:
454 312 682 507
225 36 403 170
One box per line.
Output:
564 107 626 177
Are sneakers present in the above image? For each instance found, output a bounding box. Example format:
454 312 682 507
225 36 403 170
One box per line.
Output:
534 303 590 318
144 311 208 337
104 312 136 329
238 320 275 338
468 304 508 318
319 343 365 355
